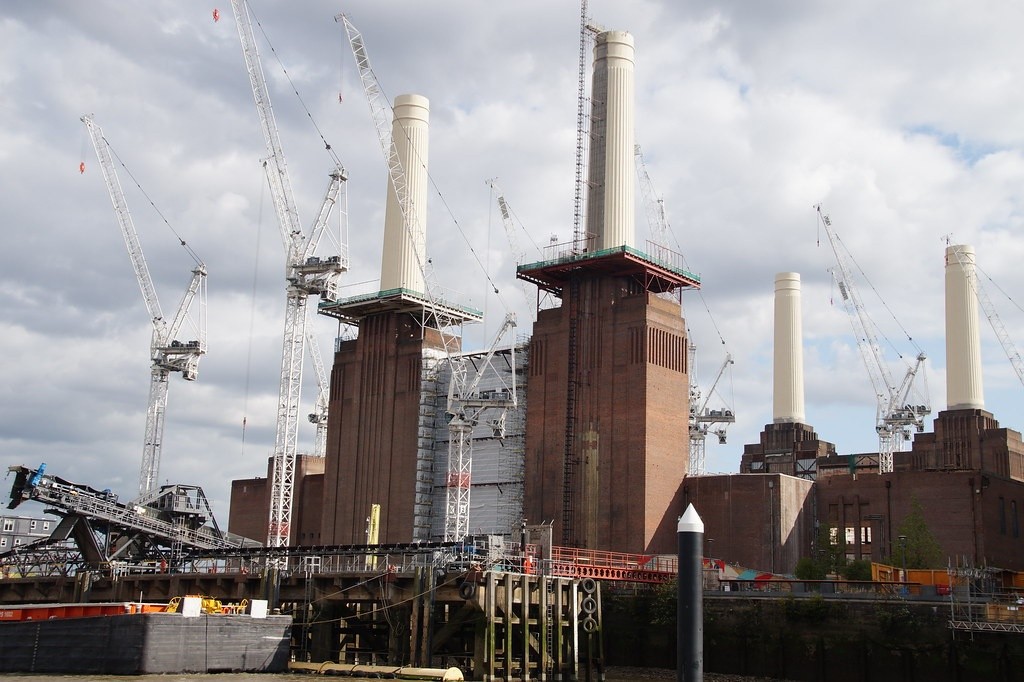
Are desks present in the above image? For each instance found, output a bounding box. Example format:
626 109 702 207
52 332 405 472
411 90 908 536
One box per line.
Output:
221 606 247 614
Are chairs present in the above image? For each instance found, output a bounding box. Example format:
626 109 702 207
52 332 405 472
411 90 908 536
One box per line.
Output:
164 597 183 613
237 599 248 614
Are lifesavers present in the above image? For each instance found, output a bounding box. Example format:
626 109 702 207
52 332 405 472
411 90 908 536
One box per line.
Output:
581 578 596 594
583 618 596 633
582 598 597 614
459 582 475 599
